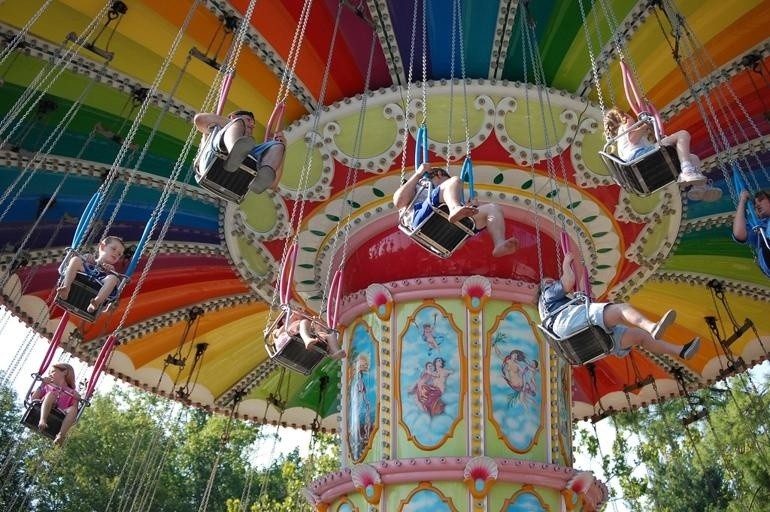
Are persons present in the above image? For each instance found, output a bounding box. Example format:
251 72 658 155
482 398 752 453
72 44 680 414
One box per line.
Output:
350 370 373 448
413 314 441 353
191 110 287 195
392 162 520 258
731 189 770 273
413 358 450 412
603 107 724 203
537 251 700 361
272 306 346 362
494 344 539 415
32 363 80 446
56 236 126 314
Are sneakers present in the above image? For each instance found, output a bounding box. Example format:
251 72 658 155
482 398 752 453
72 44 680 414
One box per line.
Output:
223 137 255 172
651 309 676 341
679 337 700 359
248 165 275 194
687 186 723 203
677 169 708 187
